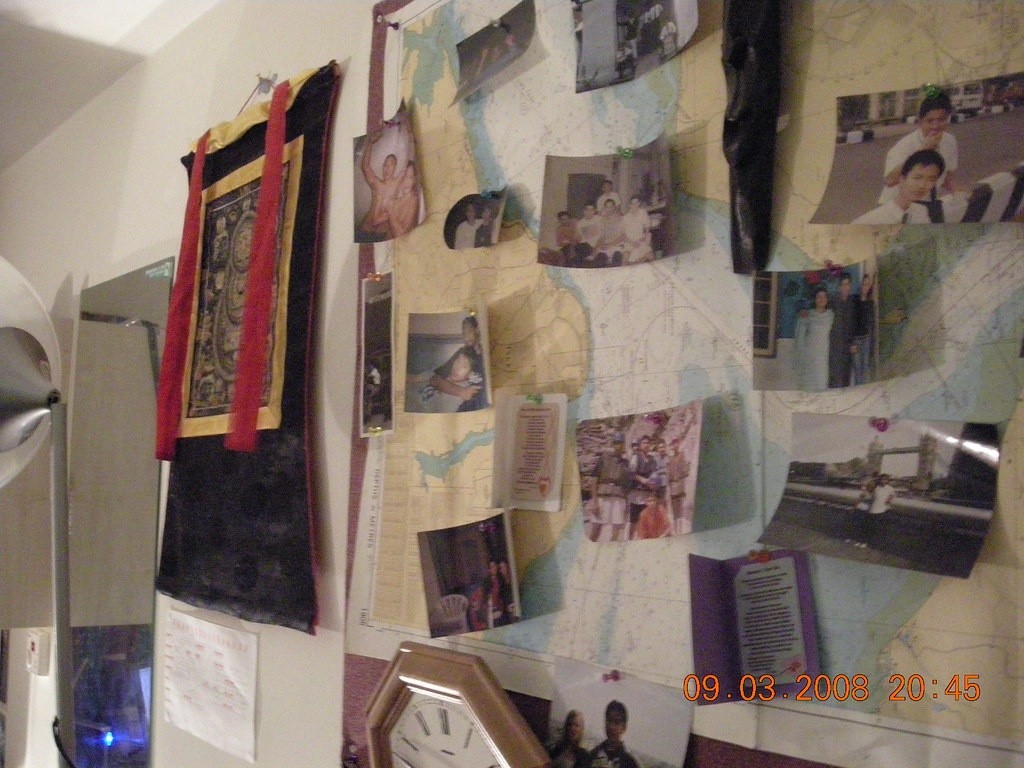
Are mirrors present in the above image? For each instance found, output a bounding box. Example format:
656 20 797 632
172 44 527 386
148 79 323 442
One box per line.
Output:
65 255 175 766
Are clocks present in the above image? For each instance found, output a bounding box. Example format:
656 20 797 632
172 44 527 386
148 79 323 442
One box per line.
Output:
364 640 553 768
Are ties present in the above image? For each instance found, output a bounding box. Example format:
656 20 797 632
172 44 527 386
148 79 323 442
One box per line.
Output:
902 212 908 224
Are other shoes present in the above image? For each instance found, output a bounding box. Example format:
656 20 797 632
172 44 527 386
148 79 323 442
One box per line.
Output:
855 542 861 547
845 539 851 543
860 543 867 548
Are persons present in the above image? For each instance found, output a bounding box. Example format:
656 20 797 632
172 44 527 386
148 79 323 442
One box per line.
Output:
372 161 418 239
878 93 959 207
793 282 851 387
558 201 601 266
406 349 480 412
851 149 945 226
852 272 874 382
556 212 578 246
595 201 620 268
430 317 486 412
455 202 484 246
595 180 619 210
355 112 416 239
593 700 641 768
590 432 690 539
476 208 493 247
500 558 516 619
488 560 500 606
550 710 590 767
474 35 516 83
622 195 654 264
792 290 833 387
846 481 876 544
853 474 895 548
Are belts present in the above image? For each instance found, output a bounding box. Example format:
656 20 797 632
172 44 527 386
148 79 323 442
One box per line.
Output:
851 335 871 340
